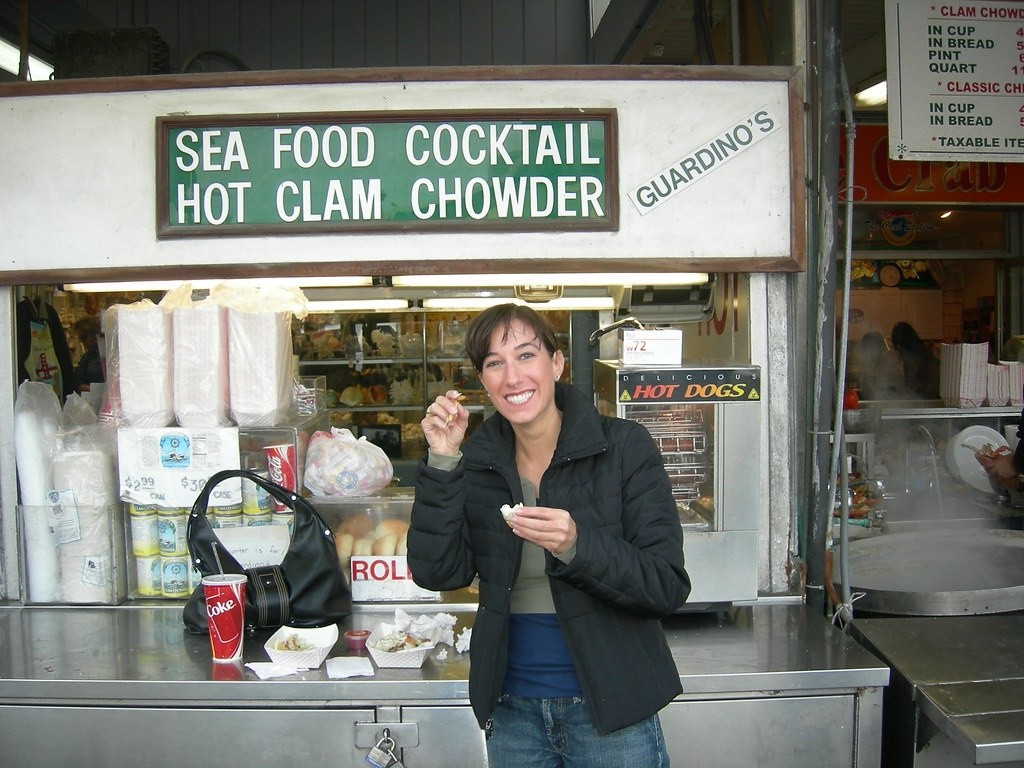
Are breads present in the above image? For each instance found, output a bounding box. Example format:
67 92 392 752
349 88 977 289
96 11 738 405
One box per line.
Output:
330 513 412 583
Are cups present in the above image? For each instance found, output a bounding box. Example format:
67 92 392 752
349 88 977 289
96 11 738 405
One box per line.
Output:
97 355 121 428
201 573 249 664
263 443 297 514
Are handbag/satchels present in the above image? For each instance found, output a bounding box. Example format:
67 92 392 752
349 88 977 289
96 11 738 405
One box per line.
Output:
183 470 353 635
304 426 393 497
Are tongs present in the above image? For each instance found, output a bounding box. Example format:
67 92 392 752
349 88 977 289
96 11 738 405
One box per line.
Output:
588 316 645 347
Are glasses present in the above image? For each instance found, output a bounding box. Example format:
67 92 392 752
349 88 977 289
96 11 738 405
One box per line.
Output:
79 335 87 342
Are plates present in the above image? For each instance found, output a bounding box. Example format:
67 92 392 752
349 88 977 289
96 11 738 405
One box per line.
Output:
944 425 1013 495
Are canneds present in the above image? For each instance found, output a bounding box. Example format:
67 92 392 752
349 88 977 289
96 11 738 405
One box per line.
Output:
129 468 295 597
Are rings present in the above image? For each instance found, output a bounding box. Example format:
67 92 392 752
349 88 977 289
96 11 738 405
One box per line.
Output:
426 402 438 413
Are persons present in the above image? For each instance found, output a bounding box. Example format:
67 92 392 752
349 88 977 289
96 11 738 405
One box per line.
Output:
73 316 113 396
985 407 1024 482
406 302 692 768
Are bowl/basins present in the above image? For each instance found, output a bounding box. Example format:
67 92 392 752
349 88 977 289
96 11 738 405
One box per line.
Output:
345 629 371 649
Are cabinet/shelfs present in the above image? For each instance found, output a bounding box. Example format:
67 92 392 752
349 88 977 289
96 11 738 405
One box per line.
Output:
910 289 945 340
0 606 891 768
836 290 909 341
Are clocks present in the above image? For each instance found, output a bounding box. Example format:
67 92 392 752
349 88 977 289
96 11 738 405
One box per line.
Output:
878 263 903 288
